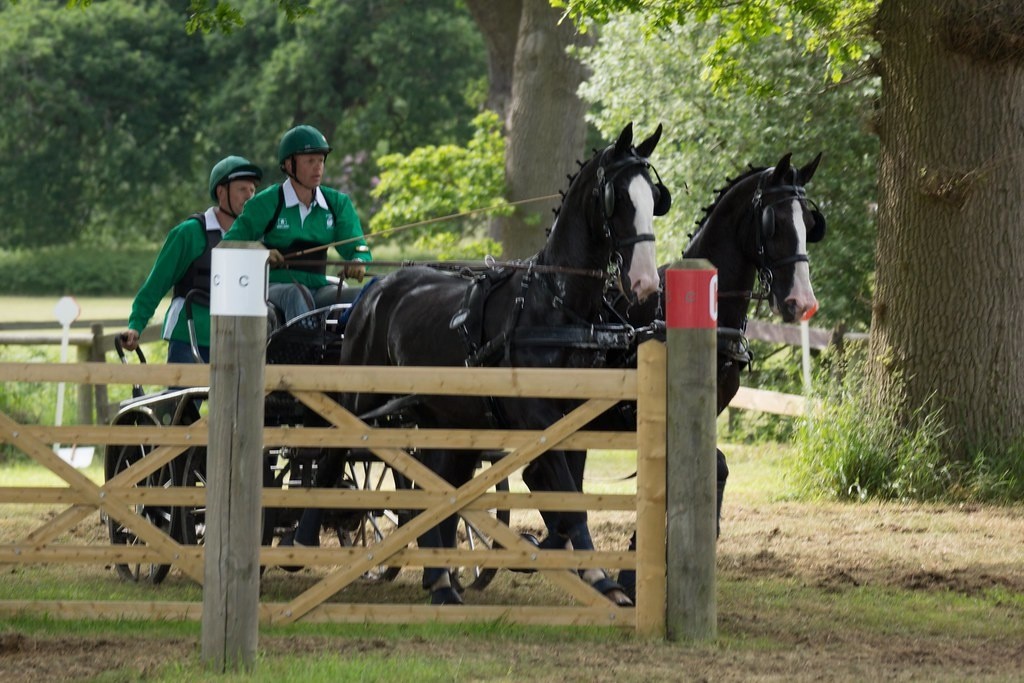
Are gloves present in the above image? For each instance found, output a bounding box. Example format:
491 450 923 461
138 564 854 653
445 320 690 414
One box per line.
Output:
338 259 366 283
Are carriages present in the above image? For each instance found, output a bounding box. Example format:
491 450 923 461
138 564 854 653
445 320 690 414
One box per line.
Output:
101 122 827 605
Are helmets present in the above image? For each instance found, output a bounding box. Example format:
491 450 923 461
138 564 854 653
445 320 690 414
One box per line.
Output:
277 125 331 168
209 156 263 202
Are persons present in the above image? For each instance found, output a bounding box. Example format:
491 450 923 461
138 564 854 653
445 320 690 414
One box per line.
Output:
120 157 266 411
222 125 373 326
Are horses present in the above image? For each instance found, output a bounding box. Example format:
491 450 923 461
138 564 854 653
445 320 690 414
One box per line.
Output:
276 120 673 609
504 151 826 614
